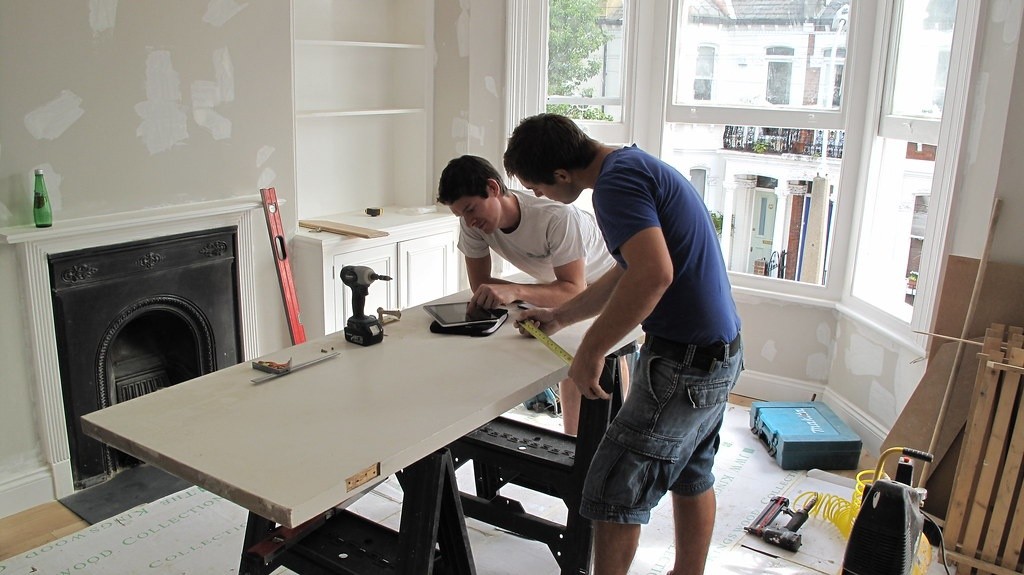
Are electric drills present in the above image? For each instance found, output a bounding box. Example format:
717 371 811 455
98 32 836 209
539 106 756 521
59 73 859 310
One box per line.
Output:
340 265 393 347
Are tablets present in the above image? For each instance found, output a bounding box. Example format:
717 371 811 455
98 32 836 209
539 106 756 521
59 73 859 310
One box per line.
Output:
423 302 499 327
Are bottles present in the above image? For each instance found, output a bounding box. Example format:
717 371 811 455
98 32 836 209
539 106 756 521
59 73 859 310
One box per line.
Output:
32 169 53 228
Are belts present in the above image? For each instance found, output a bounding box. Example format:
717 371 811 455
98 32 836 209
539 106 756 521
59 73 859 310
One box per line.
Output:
645 332 742 375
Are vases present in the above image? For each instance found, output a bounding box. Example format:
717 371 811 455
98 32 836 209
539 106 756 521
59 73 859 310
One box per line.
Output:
791 141 805 154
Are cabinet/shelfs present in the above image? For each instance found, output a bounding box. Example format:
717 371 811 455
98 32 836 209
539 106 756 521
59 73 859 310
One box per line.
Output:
294 207 472 348
290 0 436 233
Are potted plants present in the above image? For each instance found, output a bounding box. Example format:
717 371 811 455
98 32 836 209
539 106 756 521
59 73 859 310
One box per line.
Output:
909 270 918 289
752 142 772 153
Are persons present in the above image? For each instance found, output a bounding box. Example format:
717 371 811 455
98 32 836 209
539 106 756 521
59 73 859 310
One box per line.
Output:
437 155 630 435
502 113 743 575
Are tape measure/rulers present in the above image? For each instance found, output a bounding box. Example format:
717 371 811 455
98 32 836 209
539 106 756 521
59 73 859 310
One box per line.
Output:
517 319 614 396
365 207 384 217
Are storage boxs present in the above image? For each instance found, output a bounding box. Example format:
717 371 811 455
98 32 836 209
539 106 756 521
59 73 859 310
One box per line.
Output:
749 402 863 471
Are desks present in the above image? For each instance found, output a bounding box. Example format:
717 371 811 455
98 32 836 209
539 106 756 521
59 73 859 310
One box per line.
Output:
81 272 643 575
